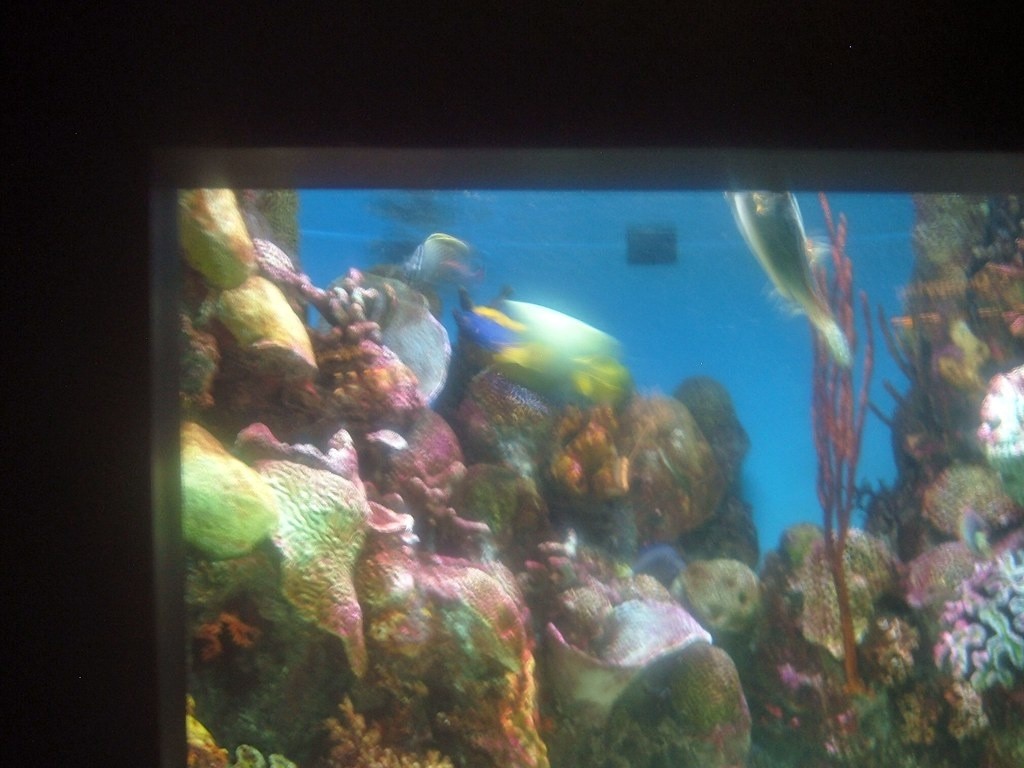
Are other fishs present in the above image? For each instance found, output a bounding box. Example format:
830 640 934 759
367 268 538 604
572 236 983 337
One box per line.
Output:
447 294 625 406
400 233 475 288
725 191 855 367
